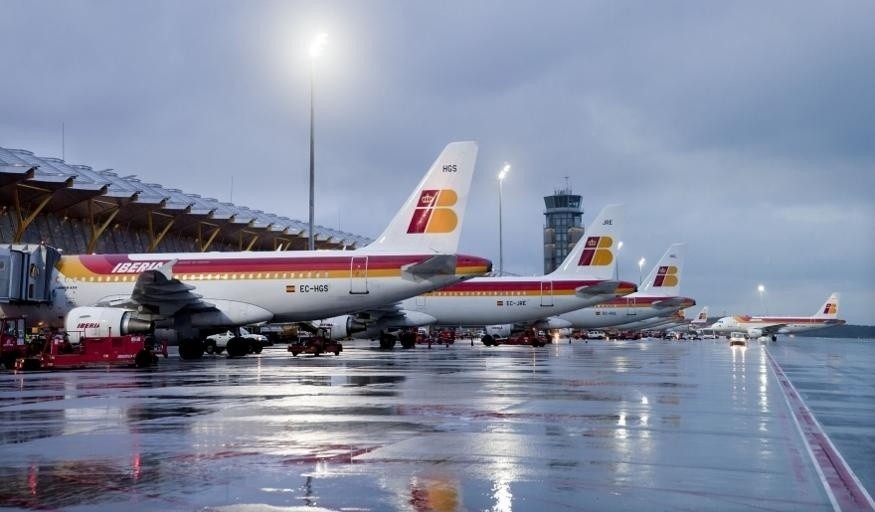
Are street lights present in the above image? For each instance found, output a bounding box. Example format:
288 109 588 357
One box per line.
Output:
296 30 334 251
489 161 516 281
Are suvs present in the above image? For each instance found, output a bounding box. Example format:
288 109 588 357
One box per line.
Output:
202 322 269 356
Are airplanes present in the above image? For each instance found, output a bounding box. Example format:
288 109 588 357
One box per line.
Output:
0 137 482 369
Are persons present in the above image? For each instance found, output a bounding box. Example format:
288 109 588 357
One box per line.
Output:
662 331 666 341
59 337 73 353
160 341 168 359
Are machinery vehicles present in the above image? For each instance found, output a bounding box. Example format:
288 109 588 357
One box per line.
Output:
287 325 344 359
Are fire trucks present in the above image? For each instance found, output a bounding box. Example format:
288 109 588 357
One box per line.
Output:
11 327 162 376
